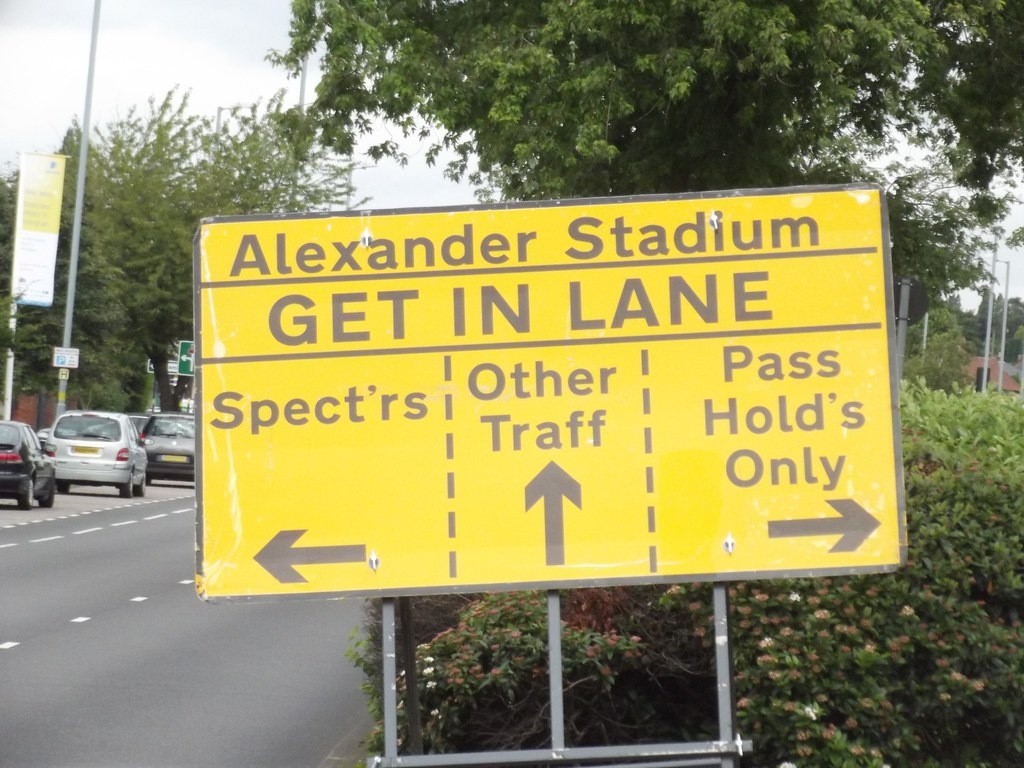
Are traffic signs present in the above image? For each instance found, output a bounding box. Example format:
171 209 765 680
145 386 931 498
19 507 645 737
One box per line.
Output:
187 181 905 610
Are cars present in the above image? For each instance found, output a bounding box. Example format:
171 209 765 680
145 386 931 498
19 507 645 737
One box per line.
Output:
128 416 149 435
0 421 55 510
46 410 147 498
36 428 51 448
137 410 195 487
